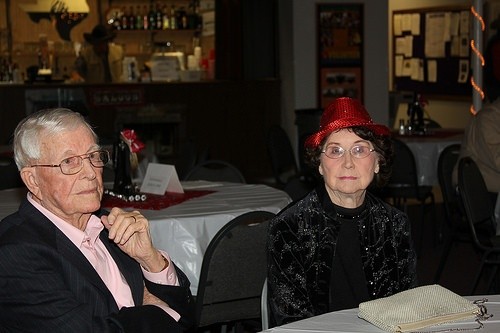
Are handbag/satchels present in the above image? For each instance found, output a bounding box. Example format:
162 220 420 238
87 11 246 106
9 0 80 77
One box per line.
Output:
358 284 494 333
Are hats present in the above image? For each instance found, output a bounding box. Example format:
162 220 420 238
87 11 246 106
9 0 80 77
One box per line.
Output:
305 97 392 152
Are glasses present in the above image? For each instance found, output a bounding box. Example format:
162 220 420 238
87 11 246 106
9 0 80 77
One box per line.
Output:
31 149 111 176
319 145 375 160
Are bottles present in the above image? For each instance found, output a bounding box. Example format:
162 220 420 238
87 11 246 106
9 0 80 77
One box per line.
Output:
411 101 424 132
112 4 202 31
399 120 405 135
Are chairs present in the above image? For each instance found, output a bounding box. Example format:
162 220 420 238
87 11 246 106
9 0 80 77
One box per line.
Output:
193 127 500 326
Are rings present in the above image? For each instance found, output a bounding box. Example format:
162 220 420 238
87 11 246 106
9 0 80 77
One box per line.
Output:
131 215 137 222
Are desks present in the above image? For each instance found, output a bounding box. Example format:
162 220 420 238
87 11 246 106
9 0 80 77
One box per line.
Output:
252 295 500 333
0 179 293 296
391 129 466 142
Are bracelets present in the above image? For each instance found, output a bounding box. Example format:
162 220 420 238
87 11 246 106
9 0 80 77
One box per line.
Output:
142 254 168 273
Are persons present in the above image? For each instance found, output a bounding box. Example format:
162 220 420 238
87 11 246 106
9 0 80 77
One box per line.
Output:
69 24 133 83
453 82 500 210
265 97 423 326
0 108 194 333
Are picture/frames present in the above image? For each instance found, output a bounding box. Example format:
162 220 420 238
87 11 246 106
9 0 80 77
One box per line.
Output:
315 3 364 114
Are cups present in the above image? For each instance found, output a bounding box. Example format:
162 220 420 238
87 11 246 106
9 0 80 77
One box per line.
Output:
188 46 209 79
